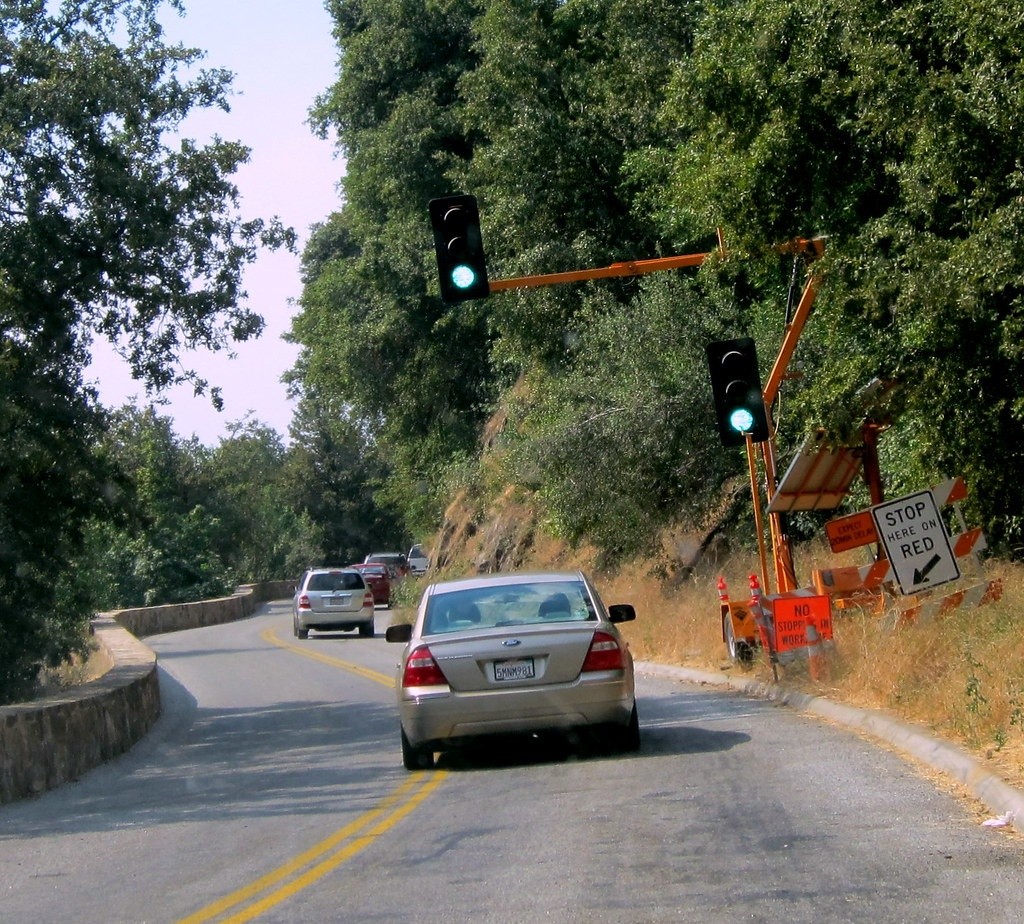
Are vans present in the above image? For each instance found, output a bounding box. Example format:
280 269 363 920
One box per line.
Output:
406 544 432 577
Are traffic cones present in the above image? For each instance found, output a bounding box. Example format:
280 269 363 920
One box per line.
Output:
805 614 831 688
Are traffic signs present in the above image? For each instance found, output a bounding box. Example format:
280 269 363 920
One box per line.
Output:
870 487 961 597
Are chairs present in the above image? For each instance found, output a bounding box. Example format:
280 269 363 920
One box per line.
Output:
539 601 569 620
448 601 480 623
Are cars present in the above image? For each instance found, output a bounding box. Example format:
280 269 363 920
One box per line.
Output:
385 568 644 771
287 567 375 640
340 563 394 609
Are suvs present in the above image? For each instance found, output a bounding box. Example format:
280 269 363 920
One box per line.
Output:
363 552 407 580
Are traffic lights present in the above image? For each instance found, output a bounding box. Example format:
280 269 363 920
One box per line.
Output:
429 194 490 304
706 336 769 448
